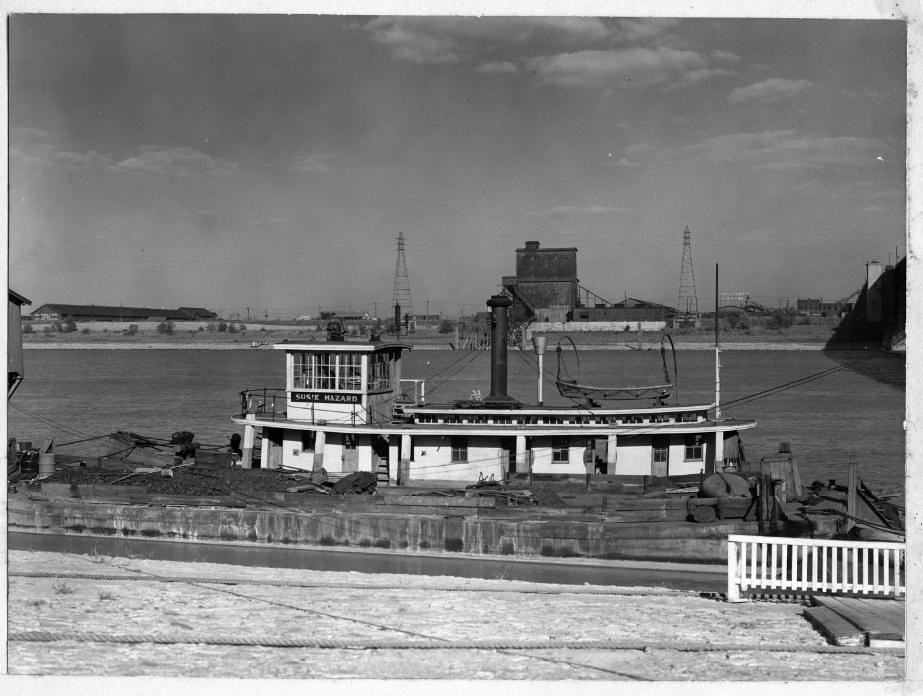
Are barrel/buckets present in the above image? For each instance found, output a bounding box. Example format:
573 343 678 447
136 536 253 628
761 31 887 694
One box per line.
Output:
37 453 57 477
21 450 39 475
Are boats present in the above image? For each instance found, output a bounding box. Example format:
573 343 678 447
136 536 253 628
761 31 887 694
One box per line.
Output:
228 290 757 495
